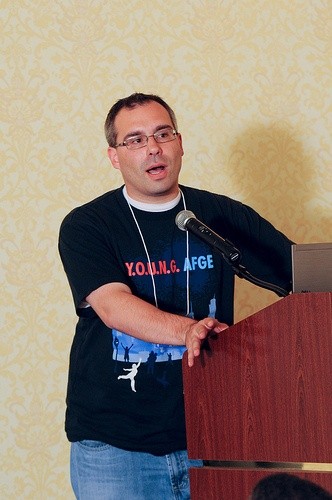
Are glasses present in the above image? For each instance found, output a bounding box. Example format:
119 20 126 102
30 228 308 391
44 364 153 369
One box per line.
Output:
113 129 178 150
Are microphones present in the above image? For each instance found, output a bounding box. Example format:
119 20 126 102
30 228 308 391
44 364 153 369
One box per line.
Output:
175 210 241 264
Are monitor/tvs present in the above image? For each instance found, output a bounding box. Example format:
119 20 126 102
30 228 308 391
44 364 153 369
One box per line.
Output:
292 243 332 292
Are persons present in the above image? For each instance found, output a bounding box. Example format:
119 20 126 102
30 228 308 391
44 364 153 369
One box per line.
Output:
59 93 296 500
249 471 332 500
